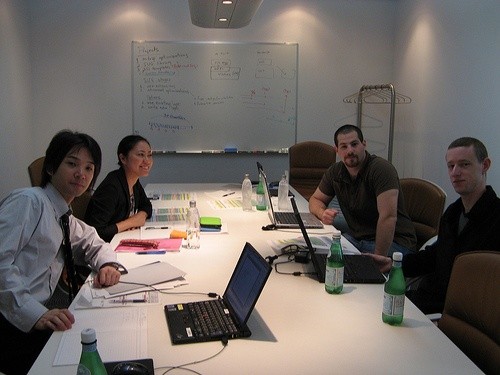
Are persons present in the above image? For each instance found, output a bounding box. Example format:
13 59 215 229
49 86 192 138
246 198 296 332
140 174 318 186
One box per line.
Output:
364 137 500 315
86 135 154 245
0 130 128 375
309 124 415 259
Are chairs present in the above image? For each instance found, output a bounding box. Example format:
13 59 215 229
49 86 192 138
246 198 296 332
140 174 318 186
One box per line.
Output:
288 140 336 202
392 178 447 249
425 250 500 375
27 154 91 220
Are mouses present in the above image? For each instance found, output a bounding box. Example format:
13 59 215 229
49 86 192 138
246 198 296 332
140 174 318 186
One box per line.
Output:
111 362 150 375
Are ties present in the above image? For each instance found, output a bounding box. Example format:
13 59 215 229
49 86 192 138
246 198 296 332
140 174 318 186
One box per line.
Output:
60 213 78 303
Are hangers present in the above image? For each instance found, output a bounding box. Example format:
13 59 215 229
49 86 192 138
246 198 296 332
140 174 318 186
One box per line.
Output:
336 113 387 155
341 83 412 104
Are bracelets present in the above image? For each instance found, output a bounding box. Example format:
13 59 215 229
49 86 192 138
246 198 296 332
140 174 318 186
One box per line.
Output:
101 263 118 270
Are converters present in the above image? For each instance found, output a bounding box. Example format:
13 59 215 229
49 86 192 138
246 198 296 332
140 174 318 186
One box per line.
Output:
293 250 311 263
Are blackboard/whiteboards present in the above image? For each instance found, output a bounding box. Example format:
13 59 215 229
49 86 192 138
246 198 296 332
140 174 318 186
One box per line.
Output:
131 40 299 155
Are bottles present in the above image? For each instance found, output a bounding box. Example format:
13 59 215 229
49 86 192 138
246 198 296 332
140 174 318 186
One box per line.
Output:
186 201 200 248
256 176 267 210
242 174 252 210
325 230 345 294
77 329 107 375
278 175 288 210
383 252 406 325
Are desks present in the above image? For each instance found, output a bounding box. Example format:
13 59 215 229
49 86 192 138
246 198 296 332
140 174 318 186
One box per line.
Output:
27 182 485 375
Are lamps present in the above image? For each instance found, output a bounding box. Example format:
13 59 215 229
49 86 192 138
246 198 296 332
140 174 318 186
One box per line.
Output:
189 0 262 29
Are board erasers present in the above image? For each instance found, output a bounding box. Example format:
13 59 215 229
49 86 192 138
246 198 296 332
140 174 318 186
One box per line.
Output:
224 147 238 153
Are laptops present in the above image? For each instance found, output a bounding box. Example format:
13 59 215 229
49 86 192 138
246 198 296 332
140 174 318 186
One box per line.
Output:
259 167 324 228
290 197 386 283
164 242 272 345
256 161 294 197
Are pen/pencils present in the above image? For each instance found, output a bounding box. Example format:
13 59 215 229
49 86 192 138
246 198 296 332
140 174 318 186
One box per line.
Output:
223 191 236 197
145 226 169 230
136 250 165 254
109 299 146 303
